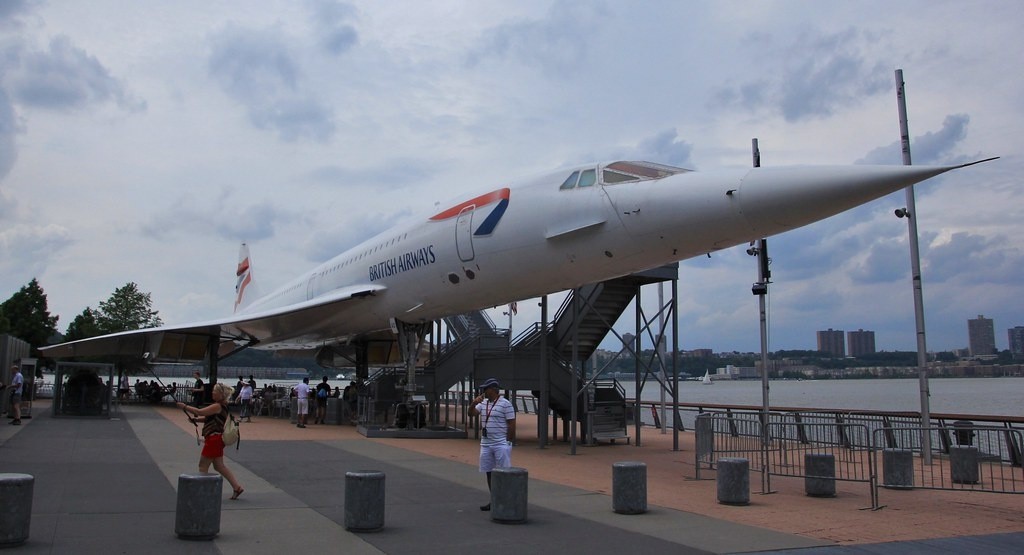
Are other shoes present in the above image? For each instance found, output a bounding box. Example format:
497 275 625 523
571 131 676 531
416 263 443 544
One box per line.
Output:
245 419 251 423
9 420 21 425
315 419 325 424
237 418 241 422
481 504 490 510
296 424 305 428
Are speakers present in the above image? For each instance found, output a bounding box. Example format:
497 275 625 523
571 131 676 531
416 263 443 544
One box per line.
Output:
752 285 767 295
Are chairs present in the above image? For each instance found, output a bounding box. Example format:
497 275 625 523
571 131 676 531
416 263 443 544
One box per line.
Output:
230 391 341 419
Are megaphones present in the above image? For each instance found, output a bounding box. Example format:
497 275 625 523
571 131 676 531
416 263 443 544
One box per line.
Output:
894 207 906 218
746 247 755 255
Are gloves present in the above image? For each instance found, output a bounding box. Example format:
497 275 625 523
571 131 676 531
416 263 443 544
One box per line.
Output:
507 441 512 451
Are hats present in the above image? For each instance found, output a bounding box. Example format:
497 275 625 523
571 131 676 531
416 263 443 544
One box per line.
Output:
241 380 250 386
479 378 500 389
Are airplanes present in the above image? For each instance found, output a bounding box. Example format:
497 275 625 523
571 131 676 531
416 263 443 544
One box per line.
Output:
38 155 1004 445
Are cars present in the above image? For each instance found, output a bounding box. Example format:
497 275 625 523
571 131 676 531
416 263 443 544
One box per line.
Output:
336 373 345 379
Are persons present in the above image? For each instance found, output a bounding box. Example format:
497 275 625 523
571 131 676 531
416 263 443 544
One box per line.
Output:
334 387 340 398
314 376 331 424
177 383 244 500
6 365 24 426
237 376 244 392
293 377 309 428
236 381 253 422
262 384 277 397
290 388 298 398
248 375 256 390
468 378 516 511
120 372 129 406
134 379 177 402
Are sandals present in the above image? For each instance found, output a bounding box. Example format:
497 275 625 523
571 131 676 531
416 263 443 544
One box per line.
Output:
230 486 243 500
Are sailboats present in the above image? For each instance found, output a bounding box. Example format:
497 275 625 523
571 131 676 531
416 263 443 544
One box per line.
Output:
702 369 715 385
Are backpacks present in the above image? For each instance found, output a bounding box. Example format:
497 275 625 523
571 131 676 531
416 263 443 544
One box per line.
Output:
317 389 326 398
218 403 239 446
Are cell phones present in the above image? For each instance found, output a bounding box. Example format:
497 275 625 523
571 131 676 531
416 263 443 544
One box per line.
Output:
143 352 149 358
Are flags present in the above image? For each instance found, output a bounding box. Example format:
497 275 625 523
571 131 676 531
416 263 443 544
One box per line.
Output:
233 244 252 313
